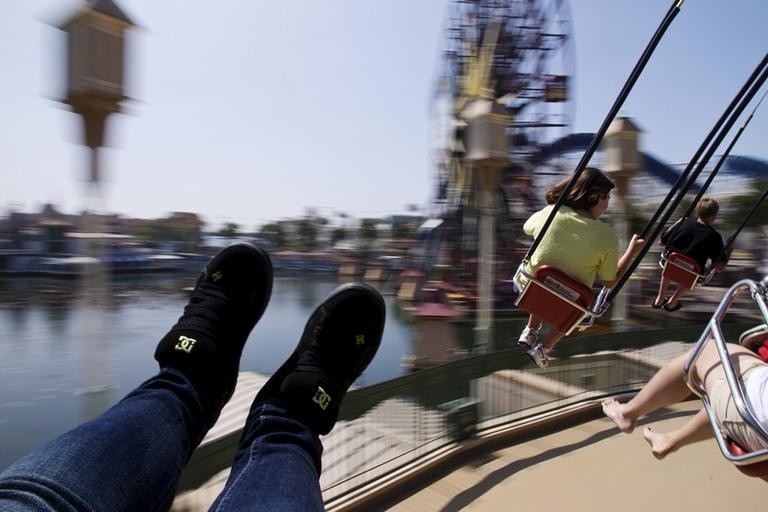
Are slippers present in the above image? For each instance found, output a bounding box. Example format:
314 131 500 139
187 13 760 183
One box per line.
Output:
652 295 683 313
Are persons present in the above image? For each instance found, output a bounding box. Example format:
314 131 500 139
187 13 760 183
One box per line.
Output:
600 336 767 462
649 198 737 310
1 241 388 512
514 167 646 368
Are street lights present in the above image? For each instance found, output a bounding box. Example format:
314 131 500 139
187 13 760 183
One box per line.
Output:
602 116 643 324
457 95 518 350
55 0 137 181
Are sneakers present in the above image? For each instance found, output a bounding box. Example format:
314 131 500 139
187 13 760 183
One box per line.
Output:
525 341 552 370
154 241 275 407
516 326 540 348
246 280 387 436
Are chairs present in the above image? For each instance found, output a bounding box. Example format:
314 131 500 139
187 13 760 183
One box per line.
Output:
685 280 768 483
660 246 716 294
513 262 606 337
